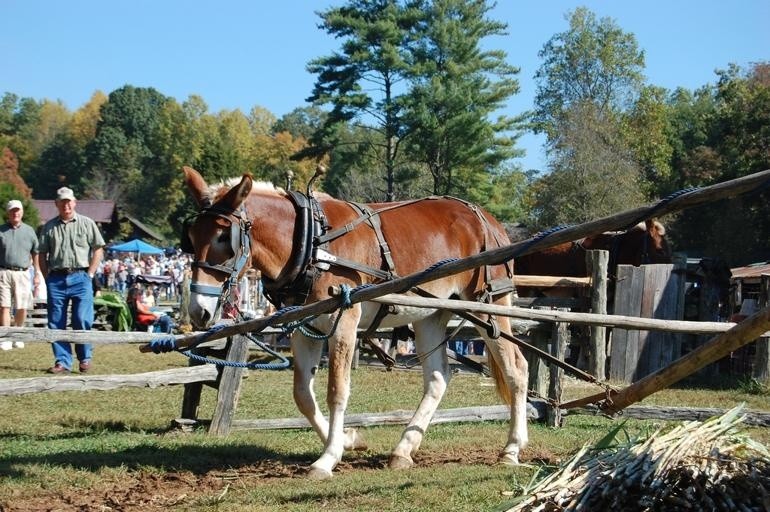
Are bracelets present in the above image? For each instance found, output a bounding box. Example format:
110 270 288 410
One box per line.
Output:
89 273 93 277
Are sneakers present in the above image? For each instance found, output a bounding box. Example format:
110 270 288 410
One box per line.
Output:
14 342 24 348
0 342 12 350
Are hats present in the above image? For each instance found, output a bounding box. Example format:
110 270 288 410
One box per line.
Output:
55 186 74 201
6 199 23 211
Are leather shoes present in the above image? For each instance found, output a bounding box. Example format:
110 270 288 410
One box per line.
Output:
47 364 66 373
79 361 91 371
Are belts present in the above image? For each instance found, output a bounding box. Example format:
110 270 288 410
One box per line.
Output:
3 265 28 271
53 269 78 273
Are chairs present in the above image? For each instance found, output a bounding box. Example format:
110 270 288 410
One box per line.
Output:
128 301 165 331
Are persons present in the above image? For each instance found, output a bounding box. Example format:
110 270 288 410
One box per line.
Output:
38 188 106 372
0 200 40 350
97 255 193 302
127 283 172 333
28 261 47 328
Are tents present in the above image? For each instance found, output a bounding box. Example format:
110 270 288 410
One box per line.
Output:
112 240 165 261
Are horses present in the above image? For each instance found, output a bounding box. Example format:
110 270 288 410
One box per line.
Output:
514 216 674 370
183 164 530 483
